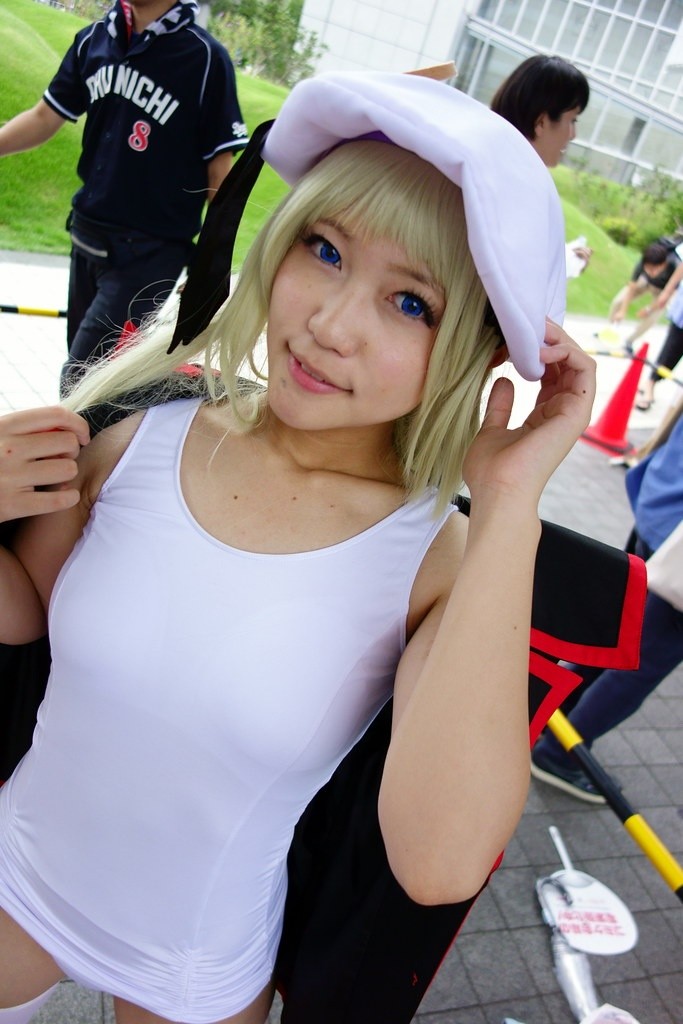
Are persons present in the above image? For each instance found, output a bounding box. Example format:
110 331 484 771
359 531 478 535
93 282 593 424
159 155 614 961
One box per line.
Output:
531 403 683 803
0 72 595 1024
637 242 683 410
610 229 682 355
0 0 251 403
491 55 590 168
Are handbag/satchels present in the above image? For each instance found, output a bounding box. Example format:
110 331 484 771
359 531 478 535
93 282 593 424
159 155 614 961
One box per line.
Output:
64 206 164 270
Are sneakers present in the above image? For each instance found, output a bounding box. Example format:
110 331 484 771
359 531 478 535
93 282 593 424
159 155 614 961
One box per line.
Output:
529 730 623 805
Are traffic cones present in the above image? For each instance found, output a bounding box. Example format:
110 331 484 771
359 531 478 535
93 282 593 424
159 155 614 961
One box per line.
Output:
576 342 653 459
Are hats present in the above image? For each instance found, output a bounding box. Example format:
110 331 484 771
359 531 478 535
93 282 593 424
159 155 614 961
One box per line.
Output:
260 70 568 382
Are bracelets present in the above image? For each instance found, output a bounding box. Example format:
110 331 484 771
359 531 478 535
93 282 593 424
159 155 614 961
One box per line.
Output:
646 305 651 312
